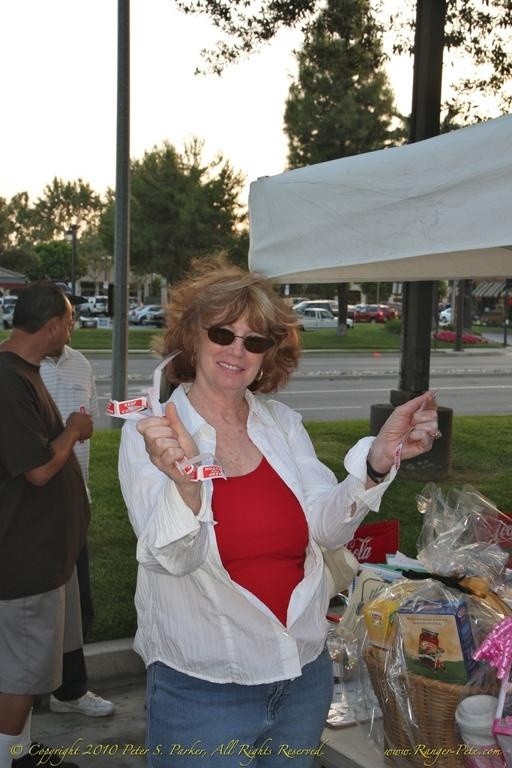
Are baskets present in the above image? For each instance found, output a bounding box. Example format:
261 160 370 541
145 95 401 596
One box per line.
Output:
361 573 511 768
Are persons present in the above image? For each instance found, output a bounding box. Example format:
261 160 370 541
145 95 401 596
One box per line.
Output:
39 281 117 718
113 267 440 768
0 280 95 768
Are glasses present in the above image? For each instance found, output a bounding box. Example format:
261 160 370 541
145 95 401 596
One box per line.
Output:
202 325 274 354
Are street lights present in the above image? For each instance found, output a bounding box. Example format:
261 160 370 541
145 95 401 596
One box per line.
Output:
65 223 81 320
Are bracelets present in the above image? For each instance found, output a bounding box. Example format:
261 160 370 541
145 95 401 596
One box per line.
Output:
365 458 392 485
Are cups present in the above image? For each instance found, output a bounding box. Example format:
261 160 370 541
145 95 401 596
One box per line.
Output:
453 694 507 768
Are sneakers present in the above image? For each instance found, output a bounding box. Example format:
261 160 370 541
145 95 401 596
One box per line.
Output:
49 691 116 717
11 741 80 768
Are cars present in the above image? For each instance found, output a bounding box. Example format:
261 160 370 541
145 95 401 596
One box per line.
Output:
0 292 452 329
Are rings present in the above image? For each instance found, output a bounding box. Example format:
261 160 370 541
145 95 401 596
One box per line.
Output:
428 429 443 442
